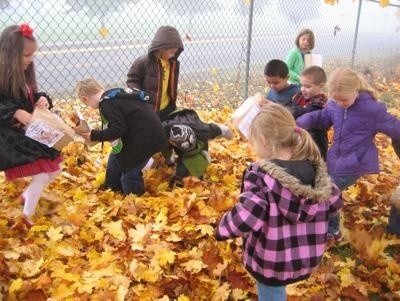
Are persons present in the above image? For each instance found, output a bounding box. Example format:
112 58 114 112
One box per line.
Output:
292 67 330 163
262 60 298 108
0 24 63 227
386 186 400 237
286 29 315 86
126 27 233 150
294 67 400 243
73 79 168 197
162 109 231 179
211 104 343 301
